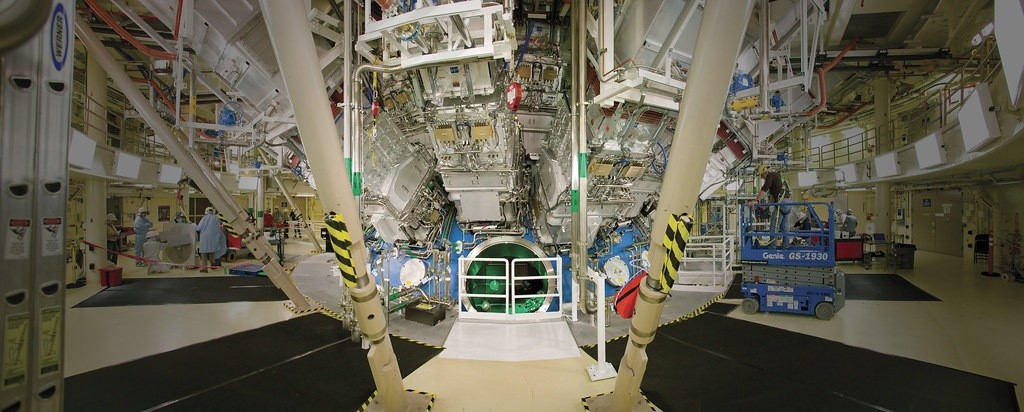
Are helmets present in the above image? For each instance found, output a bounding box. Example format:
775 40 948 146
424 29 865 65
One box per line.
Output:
146 231 159 238
138 207 147 216
274 205 279 210
107 213 117 221
204 207 213 214
758 164 768 179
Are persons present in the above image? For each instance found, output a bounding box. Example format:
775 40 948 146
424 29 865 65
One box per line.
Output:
793 211 812 247
747 170 793 251
843 209 858 237
106 213 120 266
264 206 302 239
195 207 227 272
134 207 154 267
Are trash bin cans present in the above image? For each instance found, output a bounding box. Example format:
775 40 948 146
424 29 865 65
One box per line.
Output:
894 242 918 270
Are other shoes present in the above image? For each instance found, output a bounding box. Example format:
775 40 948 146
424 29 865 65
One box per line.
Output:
200 268 208 273
137 261 146 267
148 263 153 275
211 265 217 270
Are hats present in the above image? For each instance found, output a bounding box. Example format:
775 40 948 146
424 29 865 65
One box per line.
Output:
836 209 842 214
797 212 807 221
847 209 853 214
